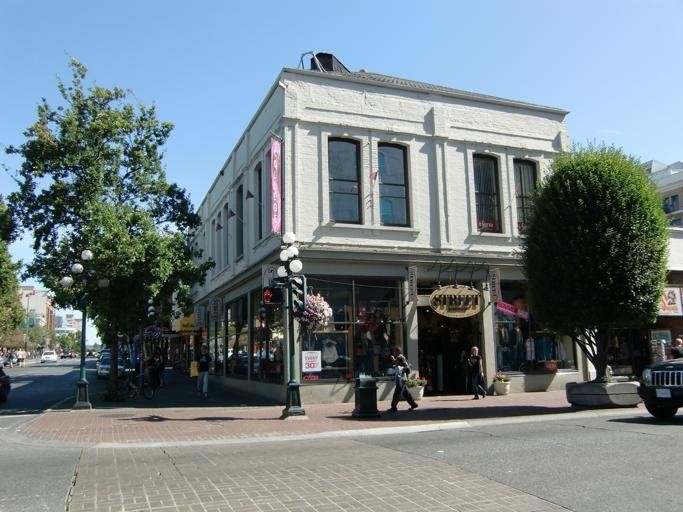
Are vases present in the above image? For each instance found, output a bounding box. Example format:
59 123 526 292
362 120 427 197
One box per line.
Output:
493 382 509 395
406 386 424 401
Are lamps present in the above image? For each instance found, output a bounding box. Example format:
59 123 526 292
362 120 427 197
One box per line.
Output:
426 259 490 291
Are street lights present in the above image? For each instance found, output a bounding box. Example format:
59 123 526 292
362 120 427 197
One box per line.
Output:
278 232 303 408
62 249 109 403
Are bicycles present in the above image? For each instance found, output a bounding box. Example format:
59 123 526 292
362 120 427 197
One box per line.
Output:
125 368 155 399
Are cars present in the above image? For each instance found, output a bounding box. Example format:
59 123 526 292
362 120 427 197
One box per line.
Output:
637 358 683 417
41 348 76 363
96 349 125 378
0 352 18 365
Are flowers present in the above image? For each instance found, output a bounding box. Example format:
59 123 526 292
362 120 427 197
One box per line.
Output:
291 291 333 343
404 376 427 388
492 370 511 384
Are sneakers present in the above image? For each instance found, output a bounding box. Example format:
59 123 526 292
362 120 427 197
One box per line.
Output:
388 408 397 412
408 403 418 411
472 397 478 400
482 391 485 398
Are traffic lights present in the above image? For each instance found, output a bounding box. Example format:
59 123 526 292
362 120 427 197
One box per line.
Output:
262 286 282 305
291 275 306 312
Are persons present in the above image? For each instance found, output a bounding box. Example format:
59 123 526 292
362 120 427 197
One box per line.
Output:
468 346 488 400
196 345 212 399
387 344 419 412
671 337 683 359
154 347 164 387
0 347 27 368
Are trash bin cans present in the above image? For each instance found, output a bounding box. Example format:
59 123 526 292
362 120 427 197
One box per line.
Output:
352 377 380 418
